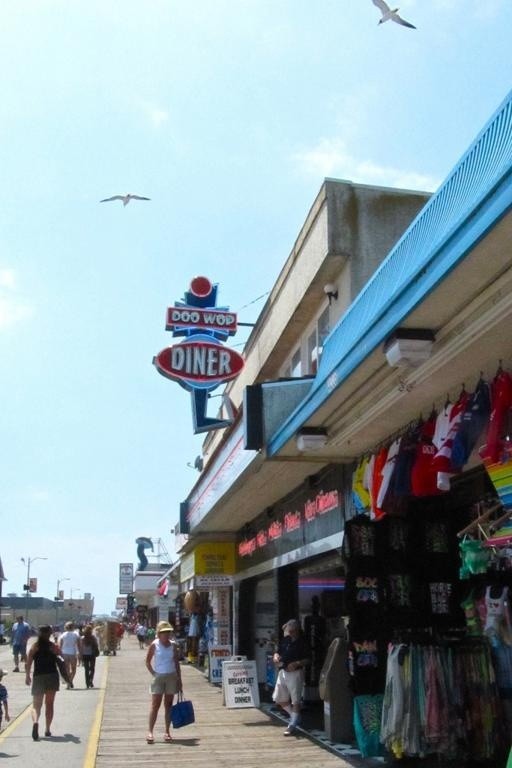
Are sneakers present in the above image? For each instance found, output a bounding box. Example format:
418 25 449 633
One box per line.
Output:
46 732 51 736
284 725 295 735
33 723 38 740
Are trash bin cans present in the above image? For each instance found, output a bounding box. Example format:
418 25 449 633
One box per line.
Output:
323 693 354 744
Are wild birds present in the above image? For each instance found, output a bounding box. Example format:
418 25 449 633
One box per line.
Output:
99 194 152 207
369 0 417 30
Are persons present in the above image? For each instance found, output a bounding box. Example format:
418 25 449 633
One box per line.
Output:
25 624 75 741
1 667 12 735
79 624 100 689
271 619 312 737
10 615 39 673
57 621 82 690
145 620 183 745
116 621 148 650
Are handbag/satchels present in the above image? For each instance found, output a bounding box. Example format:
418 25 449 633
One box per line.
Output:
171 701 194 728
92 645 99 657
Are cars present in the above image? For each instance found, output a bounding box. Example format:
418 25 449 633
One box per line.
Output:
0 623 67 637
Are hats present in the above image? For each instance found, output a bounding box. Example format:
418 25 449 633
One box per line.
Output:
0 669 8 677
283 619 299 636
157 621 174 632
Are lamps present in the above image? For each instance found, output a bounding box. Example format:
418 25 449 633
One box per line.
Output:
382 328 436 369
295 426 329 452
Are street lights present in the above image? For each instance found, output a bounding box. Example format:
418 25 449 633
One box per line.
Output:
54 576 71 625
69 587 83 622
21 555 48 627
